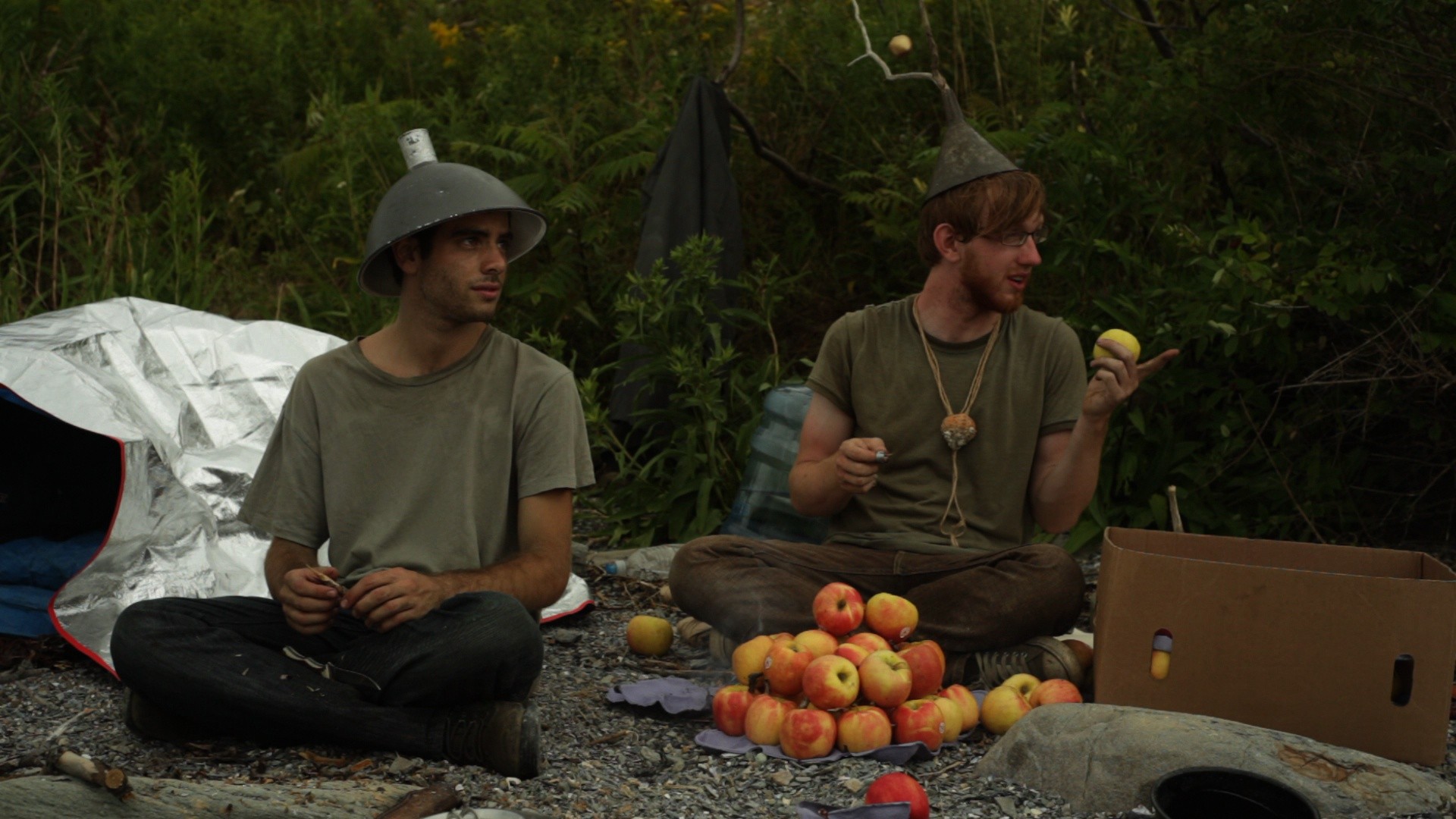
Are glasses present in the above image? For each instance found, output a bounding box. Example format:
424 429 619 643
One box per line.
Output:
974 226 1050 247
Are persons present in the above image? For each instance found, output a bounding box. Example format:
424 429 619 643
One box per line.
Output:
111 162 595 780
668 122 1178 682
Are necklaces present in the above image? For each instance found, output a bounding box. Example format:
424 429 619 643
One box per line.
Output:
912 295 1002 551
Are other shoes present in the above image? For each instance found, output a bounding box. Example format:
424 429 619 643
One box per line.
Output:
445 700 541 779
124 693 232 741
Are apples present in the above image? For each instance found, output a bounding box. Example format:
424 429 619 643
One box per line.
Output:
1093 330 1140 364
628 615 673 658
1150 650 1170 678
713 582 1093 756
889 35 911 58
865 772 929 819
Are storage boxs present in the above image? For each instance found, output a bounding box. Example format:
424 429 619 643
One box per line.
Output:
1094 525 1456 767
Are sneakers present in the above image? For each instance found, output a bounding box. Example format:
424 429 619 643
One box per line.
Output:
969 634 1085 687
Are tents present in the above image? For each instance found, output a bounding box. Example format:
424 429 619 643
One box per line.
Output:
0 296 594 683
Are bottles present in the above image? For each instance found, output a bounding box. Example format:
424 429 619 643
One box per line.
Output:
721 383 824 546
606 544 683 580
